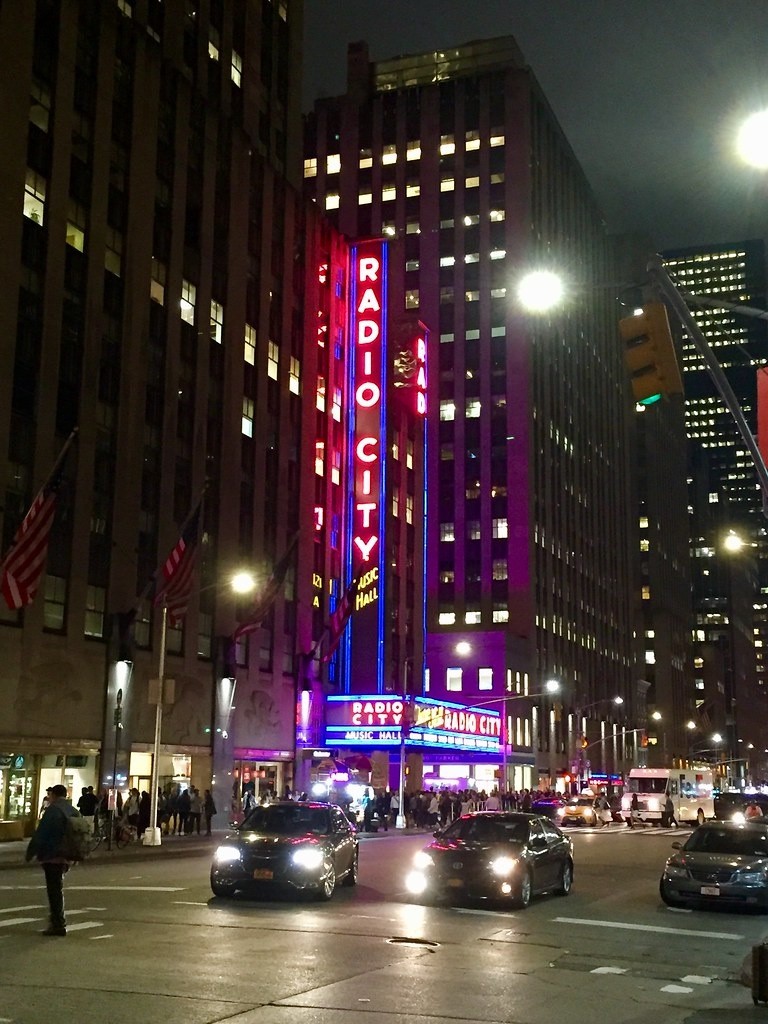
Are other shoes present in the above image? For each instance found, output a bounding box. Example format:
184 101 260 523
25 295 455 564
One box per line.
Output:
43 925 67 936
205 832 212 836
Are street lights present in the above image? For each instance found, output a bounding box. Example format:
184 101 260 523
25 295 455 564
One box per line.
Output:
142 570 254 846
394 682 562 829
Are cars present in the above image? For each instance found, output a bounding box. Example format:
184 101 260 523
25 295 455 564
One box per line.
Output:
409 809 574 908
560 797 609 826
210 801 361 901
659 821 768 908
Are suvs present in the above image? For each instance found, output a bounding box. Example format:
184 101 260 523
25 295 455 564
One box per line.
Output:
619 768 716 826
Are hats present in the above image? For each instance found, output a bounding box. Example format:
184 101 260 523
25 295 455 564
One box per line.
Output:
46 787 52 792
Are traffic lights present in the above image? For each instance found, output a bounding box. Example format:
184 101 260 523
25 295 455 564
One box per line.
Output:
641 735 650 748
616 296 687 405
581 736 588 748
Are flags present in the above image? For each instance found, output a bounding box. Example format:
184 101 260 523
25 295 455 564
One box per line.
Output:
322 585 356 664
693 703 711 733
0 460 64 607
234 546 293 643
150 503 202 625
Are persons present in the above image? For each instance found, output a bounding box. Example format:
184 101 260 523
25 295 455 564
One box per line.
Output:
259 785 308 804
77 786 217 842
745 800 763 818
629 794 646 829
375 788 570 831
26 785 82 934
600 792 610 828
243 789 256 817
662 792 678 828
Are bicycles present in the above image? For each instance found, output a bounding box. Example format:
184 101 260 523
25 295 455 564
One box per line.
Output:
88 815 136 851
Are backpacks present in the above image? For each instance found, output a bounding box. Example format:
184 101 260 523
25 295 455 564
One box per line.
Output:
51 803 93 861
